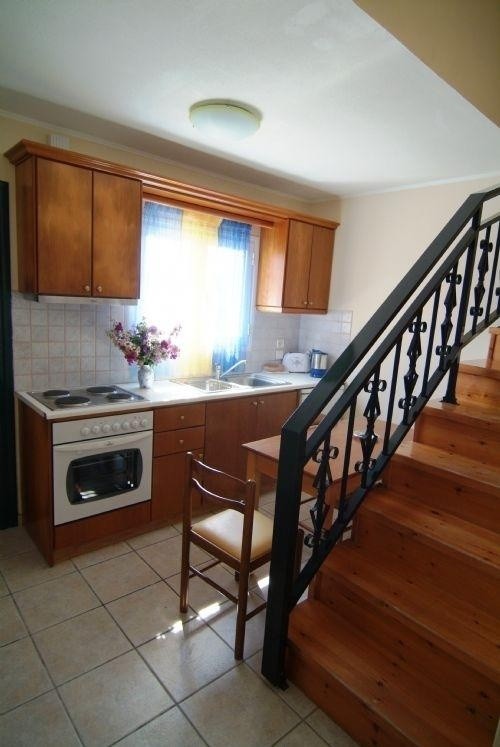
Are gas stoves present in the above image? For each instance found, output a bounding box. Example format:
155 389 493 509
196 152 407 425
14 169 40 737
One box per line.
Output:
28 384 142 410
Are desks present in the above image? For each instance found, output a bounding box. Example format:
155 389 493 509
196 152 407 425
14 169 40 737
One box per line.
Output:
243 414 415 547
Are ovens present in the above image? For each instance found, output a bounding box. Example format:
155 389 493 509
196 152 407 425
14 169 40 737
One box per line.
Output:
51 411 152 528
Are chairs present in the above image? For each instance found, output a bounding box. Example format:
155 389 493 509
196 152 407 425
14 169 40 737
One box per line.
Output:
179 451 305 664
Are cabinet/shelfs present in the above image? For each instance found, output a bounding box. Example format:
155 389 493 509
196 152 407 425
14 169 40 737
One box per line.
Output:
151 401 207 522
260 214 339 315
4 139 141 309
207 390 301 512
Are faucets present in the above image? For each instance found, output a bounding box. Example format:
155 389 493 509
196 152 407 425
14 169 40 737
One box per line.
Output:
216 359 247 380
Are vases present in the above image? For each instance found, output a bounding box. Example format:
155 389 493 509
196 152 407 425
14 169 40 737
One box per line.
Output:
138 365 154 390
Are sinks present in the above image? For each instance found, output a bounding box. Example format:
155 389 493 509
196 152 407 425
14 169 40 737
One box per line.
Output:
182 379 235 393
229 377 276 389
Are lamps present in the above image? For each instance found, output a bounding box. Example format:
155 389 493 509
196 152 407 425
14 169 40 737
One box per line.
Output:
188 99 261 150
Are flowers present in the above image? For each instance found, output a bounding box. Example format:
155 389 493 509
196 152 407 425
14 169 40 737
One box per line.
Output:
105 315 183 367
